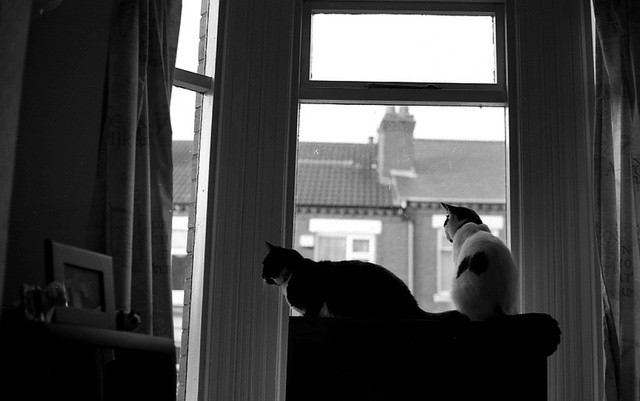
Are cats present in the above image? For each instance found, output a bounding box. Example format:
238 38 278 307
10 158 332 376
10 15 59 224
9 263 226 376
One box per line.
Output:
261 240 471 329
439 202 520 328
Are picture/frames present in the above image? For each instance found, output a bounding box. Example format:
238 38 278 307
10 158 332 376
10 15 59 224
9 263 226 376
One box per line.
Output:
45 240 117 330
